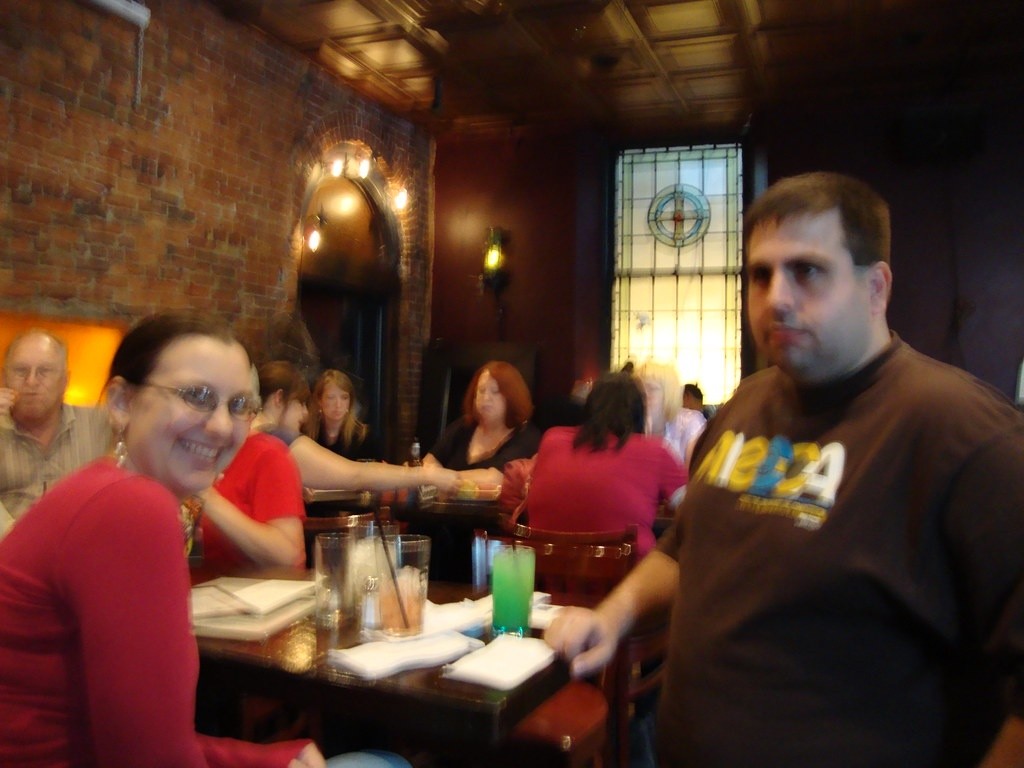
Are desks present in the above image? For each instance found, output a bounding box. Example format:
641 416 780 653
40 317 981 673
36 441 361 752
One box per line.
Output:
288 481 509 580
188 562 584 768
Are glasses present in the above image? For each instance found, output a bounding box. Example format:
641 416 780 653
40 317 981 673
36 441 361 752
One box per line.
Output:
137 381 263 422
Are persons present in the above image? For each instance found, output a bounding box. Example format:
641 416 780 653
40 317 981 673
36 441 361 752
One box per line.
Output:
525 373 689 768
544 172 1024 768
398 360 540 584
636 359 712 470
185 337 310 745
249 361 464 570
0 311 415 768
0 329 116 538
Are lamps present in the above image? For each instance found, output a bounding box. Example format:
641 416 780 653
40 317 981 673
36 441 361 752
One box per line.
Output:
479 229 510 296
303 148 382 252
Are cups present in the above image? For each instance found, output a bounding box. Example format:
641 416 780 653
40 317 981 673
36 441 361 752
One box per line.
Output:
490 546 536 637
373 535 431 637
314 531 361 663
348 520 401 630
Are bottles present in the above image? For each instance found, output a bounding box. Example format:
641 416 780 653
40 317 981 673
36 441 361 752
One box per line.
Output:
409 442 421 467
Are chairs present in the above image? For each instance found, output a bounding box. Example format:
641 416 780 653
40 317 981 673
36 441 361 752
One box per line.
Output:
507 521 668 768
